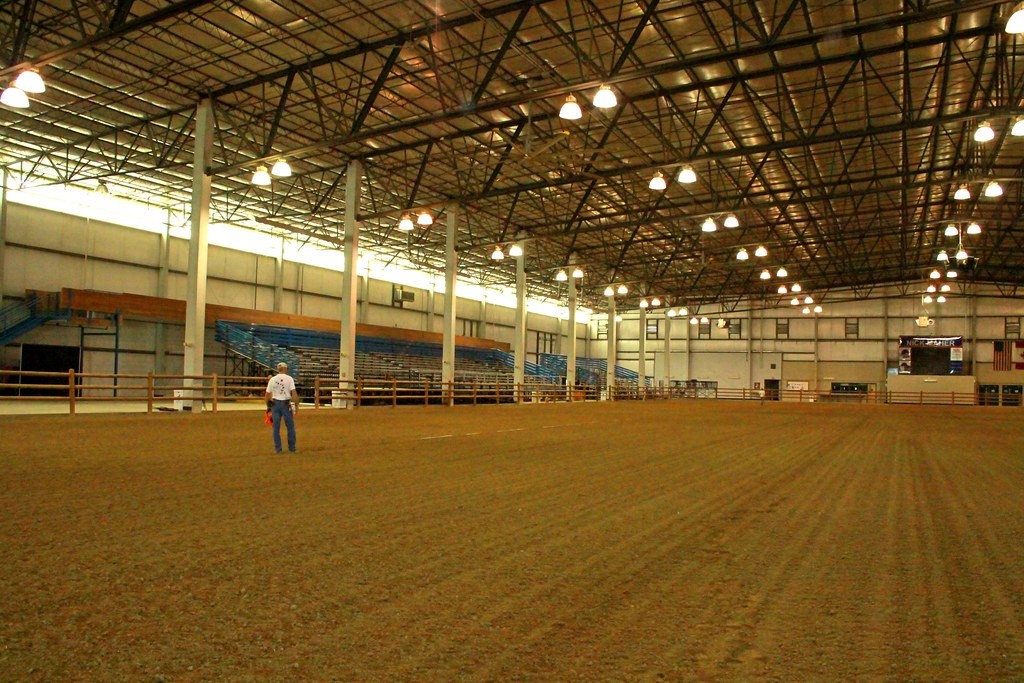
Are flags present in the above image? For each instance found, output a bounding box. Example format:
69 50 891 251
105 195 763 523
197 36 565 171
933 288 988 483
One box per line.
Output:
1011 340 1024 363
994 341 1011 371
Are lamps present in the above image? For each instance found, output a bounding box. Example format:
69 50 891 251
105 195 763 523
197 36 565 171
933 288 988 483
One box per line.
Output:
954 184 970 200
398 211 988 325
648 172 666 190
984 181 1003 197
1011 116 1024 136
0 67 46 108
1003 1 1024 34
678 165 696 184
252 158 292 186
974 121 994 142
558 94 582 120
592 84 617 109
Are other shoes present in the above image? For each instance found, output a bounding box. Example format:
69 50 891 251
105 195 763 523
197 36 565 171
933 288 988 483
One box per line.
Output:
289 450 298 454
272 450 282 455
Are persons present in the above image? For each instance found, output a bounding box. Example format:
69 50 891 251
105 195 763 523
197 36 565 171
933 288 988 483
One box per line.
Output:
759 388 765 400
265 363 299 453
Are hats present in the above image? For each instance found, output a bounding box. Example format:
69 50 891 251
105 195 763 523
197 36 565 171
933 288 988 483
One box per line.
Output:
277 362 288 369
